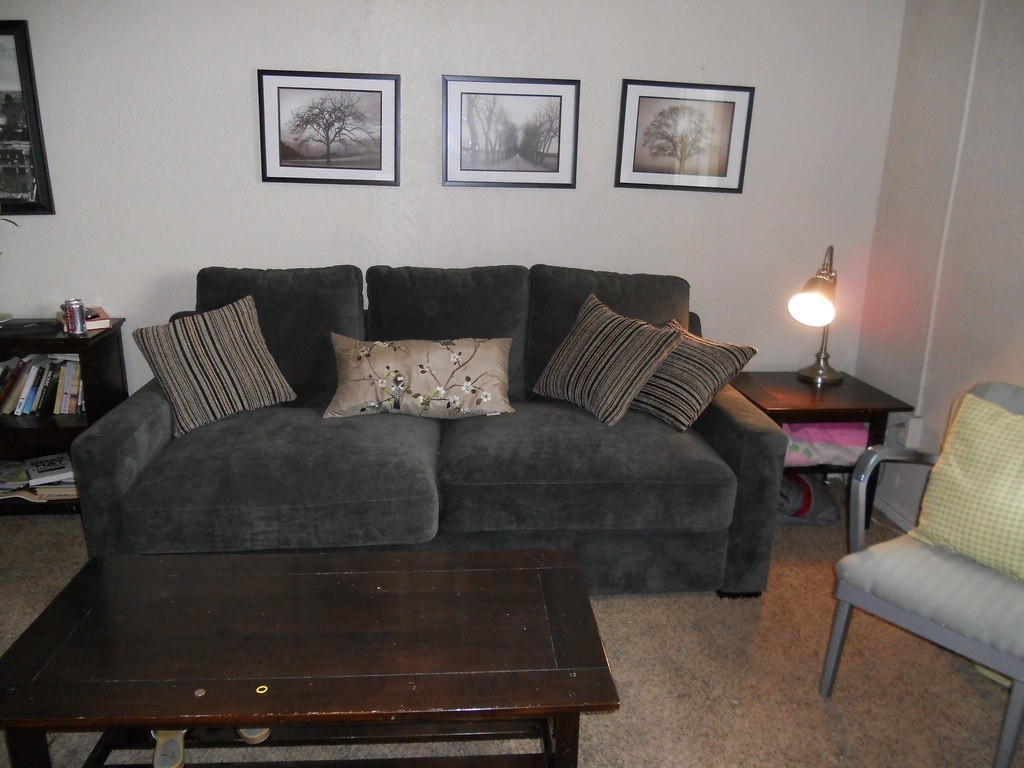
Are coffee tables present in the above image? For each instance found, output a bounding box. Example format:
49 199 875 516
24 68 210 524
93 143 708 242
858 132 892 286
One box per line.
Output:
0 546 620 768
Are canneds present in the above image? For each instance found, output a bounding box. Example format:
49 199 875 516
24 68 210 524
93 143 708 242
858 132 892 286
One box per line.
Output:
65 299 87 335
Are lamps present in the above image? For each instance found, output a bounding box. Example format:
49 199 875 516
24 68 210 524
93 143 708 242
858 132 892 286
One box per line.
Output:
787 244 845 384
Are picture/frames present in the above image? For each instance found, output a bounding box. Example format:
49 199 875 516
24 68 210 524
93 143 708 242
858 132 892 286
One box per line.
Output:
257 69 401 187
613 78 756 194
442 74 581 190
0 19 57 216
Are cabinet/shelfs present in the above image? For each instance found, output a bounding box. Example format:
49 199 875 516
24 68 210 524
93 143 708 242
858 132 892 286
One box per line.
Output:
0 318 131 517
730 372 914 529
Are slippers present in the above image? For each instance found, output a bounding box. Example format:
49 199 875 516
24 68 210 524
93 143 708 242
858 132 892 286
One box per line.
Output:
150 730 189 768
237 727 270 744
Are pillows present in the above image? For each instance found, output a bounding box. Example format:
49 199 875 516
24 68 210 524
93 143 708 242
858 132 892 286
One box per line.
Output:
907 393 1023 583
533 293 684 425
131 294 298 439
322 331 516 418
628 318 758 433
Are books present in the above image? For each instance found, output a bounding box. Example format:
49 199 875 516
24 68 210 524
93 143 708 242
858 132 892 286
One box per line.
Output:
57 306 111 332
0 453 77 503
0 354 85 416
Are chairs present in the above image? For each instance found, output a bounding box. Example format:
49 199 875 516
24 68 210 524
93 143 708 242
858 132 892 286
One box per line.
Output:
819 379 1024 768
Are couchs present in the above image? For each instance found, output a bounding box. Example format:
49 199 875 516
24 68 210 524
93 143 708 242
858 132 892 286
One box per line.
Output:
69 264 790 597
